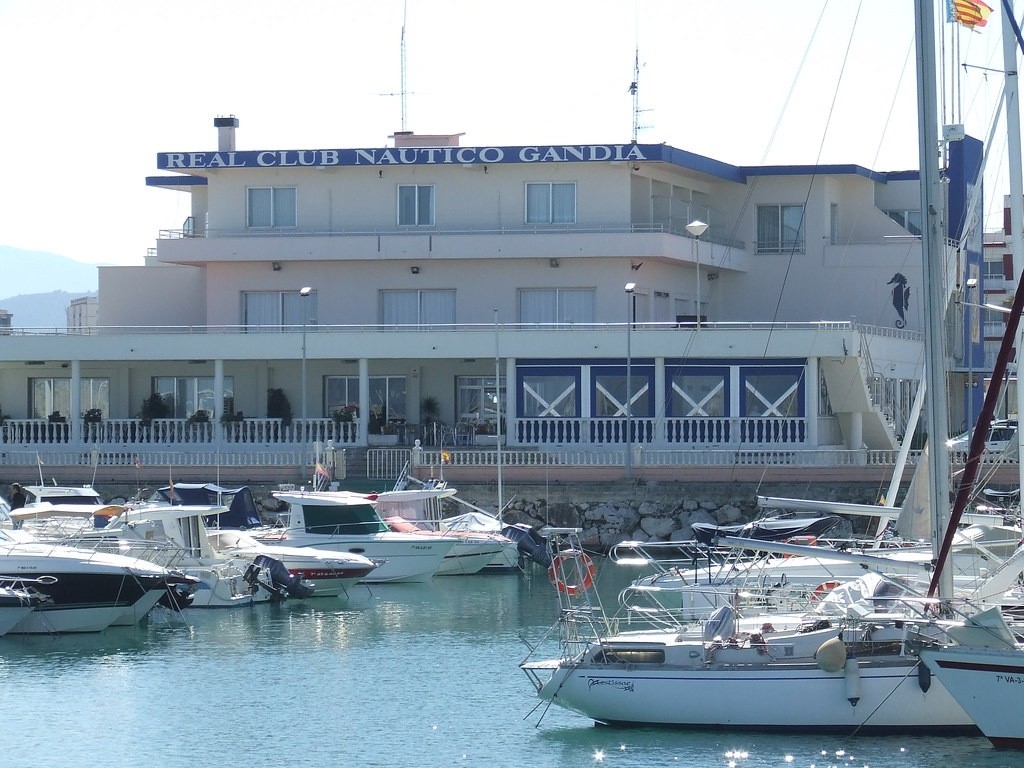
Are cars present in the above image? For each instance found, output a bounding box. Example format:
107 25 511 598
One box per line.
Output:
945 419 1019 462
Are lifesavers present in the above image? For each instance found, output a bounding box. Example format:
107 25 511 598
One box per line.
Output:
548 549 595 595
811 581 844 603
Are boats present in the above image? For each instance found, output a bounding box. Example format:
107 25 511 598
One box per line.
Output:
1 439 539 638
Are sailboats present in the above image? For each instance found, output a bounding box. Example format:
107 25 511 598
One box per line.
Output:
521 1 1024 749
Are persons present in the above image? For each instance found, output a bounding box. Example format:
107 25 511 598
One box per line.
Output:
10 483 25 529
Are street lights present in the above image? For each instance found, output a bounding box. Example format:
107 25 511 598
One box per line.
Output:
967 279 977 454
685 220 709 331
298 286 312 482
623 283 637 478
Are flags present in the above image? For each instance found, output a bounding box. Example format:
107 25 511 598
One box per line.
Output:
946 0 994 35
136 456 140 468
441 450 450 462
317 462 329 477
